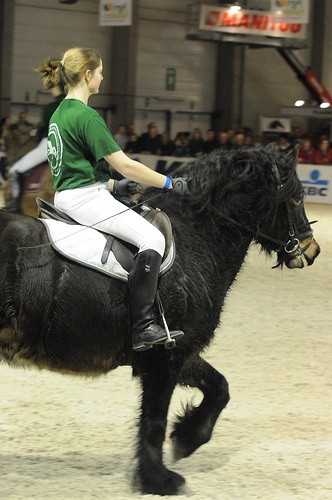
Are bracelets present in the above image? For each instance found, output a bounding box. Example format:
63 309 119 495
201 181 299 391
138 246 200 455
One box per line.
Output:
164 175 171 190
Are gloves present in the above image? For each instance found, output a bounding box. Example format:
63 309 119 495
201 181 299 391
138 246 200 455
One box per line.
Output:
171 176 194 197
112 178 139 197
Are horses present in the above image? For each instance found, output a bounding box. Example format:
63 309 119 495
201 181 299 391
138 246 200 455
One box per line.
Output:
0 141 320 497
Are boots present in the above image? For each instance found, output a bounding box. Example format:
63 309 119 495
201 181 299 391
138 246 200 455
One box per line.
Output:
125 249 186 350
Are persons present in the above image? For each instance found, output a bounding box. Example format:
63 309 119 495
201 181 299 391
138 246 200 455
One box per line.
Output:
39 46 184 351
0 79 65 216
0 108 37 188
114 120 332 165
